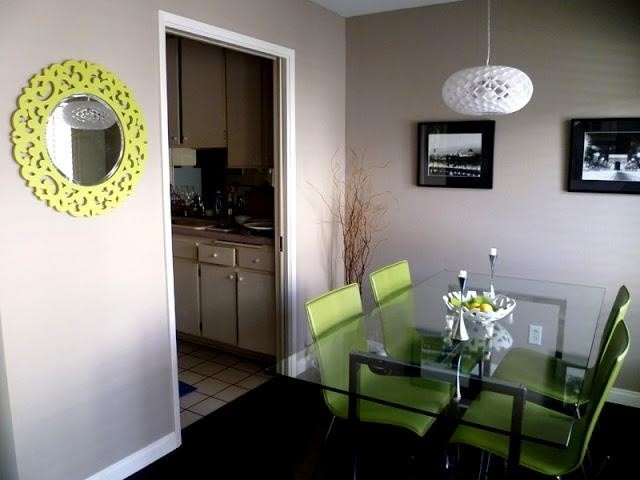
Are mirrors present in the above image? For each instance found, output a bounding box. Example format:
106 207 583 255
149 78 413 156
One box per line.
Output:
9 59 147 217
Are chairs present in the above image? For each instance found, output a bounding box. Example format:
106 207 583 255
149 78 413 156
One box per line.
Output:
449 318 631 479
369 260 487 379
491 285 631 466
305 283 456 442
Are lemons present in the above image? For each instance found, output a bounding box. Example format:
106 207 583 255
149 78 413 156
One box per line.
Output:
479 304 492 312
470 302 479 308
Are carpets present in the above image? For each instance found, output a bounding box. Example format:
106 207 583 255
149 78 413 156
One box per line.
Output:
126 360 638 480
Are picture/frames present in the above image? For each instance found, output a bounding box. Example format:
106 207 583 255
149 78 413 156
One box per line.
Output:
566 117 640 195
416 120 495 189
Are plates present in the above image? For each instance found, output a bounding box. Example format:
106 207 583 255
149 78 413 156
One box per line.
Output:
244 221 273 230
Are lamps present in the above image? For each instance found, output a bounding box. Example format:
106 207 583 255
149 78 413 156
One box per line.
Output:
441 0 533 116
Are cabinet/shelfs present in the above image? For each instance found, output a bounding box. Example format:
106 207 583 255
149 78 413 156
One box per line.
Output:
166 35 274 171
171 234 275 360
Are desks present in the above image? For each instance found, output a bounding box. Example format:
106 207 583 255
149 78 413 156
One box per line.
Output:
262 270 606 480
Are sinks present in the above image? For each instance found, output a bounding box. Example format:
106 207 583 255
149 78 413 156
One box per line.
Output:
171 217 217 230
